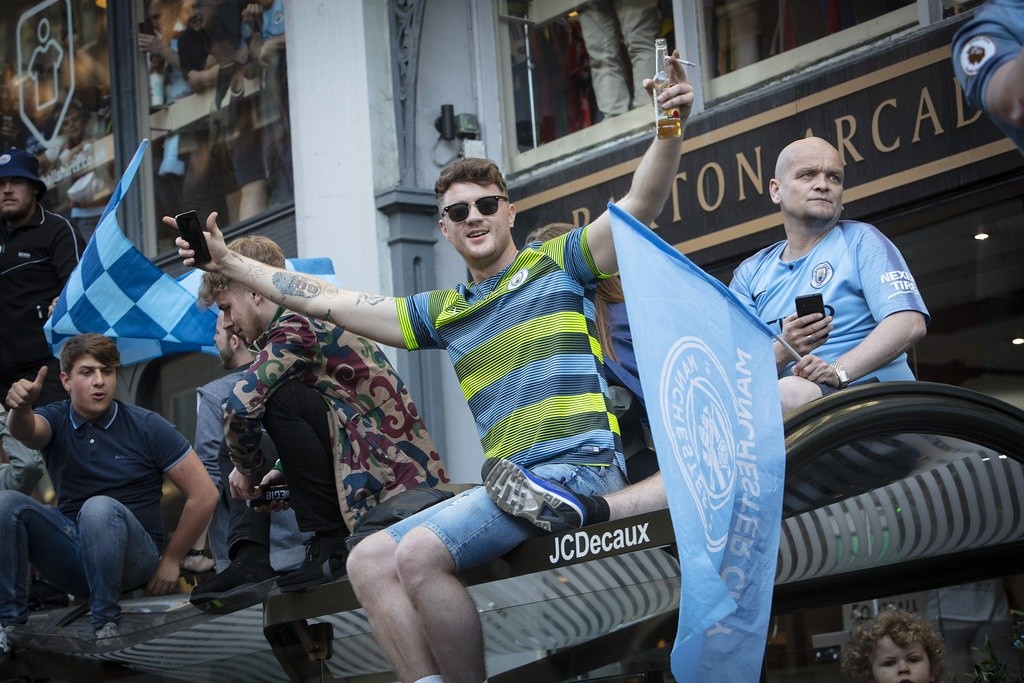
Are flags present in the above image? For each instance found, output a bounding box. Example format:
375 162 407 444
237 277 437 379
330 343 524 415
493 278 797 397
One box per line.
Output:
42 137 220 367
604 201 784 683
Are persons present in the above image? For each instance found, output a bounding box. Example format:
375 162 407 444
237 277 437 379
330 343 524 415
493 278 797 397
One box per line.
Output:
0 0 111 155
951 0 1024 157
183 236 454 615
39 103 110 212
840 611 946 683
138 0 293 231
163 50 694 683
577 0 663 120
0 151 87 612
0 335 219 653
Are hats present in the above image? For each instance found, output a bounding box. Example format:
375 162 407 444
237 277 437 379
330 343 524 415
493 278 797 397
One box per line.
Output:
0 147 48 201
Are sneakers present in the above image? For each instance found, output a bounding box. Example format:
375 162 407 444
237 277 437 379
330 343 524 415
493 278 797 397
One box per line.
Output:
275 535 350 592
96 620 123 653
189 560 275 615
481 457 588 534
0 623 17 658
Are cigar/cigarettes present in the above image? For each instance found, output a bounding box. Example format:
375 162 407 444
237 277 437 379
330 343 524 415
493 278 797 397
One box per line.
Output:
664 55 696 67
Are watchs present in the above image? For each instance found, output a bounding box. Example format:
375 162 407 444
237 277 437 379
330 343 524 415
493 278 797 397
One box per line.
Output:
830 363 849 389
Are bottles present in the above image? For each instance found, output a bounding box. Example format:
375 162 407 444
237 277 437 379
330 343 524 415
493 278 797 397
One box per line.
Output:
651 38 682 140
149 54 167 107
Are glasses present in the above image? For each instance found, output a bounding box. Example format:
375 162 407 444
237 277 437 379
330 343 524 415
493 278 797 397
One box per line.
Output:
441 195 511 223
179 2 204 18
148 13 161 24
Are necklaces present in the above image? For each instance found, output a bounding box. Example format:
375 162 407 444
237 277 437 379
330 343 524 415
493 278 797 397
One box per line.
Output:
481 137 930 533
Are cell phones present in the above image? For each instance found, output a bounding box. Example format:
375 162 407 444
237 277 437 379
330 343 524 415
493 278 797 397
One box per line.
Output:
138 20 155 37
175 211 212 267
794 292 829 339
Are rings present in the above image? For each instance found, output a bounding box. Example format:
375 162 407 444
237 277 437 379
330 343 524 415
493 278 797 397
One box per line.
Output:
808 335 813 343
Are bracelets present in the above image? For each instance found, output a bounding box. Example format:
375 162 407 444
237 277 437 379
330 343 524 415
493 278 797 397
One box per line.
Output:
187 549 206 556
